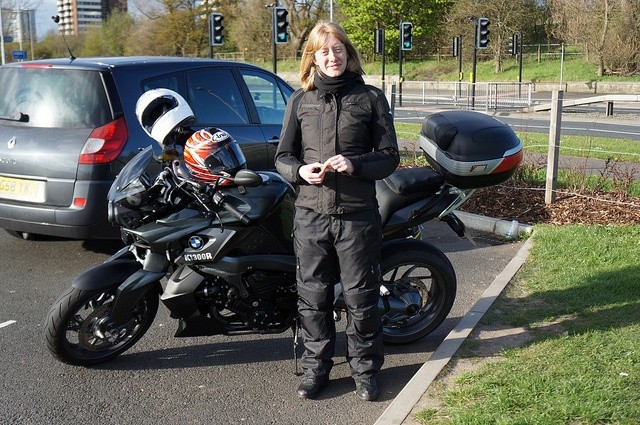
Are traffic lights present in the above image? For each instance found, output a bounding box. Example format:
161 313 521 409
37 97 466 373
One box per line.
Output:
273 7 290 44
508 33 518 55
453 36 459 57
478 18 491 48
373 28 382 54
209 13 225 46
402 21 412 50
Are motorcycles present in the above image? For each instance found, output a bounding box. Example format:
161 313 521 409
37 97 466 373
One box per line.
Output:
43 88 523 366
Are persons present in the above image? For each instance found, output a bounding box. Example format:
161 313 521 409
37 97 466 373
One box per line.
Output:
273 19 402 403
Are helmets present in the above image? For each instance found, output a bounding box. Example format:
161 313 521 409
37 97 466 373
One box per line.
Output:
136 89 195 145
184 126 246 188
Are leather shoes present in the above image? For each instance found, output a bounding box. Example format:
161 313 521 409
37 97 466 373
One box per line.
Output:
355 379 378 400
298 374 329 399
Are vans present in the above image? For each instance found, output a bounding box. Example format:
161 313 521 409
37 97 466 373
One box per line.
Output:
0 15 296 239
